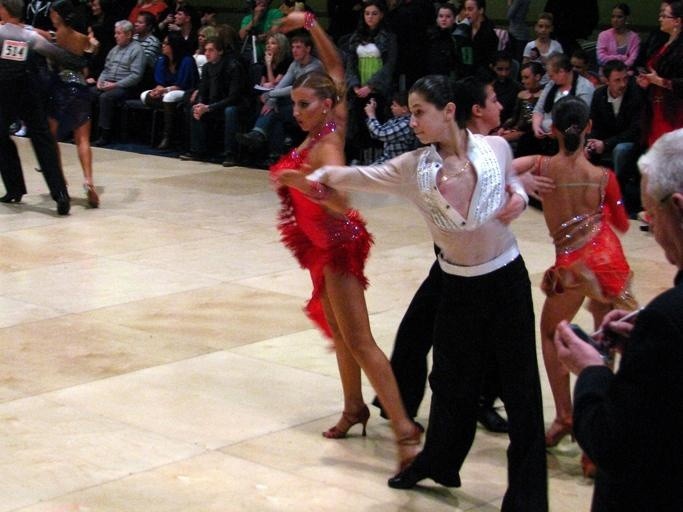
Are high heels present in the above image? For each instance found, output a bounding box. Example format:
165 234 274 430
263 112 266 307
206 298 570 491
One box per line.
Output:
83 184 99 208
583 455 596 479
546 418 575 447
322 404 369 439
397 421 424 472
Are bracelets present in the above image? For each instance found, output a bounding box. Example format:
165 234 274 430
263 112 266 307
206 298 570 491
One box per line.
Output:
305 181 332 200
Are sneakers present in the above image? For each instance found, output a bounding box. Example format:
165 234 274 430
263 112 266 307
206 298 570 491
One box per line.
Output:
14 126 27 136
236 131 261 154
180 150 200 161
222 153 234 165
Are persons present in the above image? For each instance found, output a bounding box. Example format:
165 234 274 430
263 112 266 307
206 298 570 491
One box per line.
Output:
0 0 100 215
307 75 550 511
18 0 99 208
15 0 57 136
343 1 683 218
513 96 637 479
91 1 327 166
554 128 683 512
373 77 555 431
268 13 424 471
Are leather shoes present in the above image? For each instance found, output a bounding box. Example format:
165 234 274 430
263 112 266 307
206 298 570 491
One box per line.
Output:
157 137 172 147
91 137 105 146
389 463 435 489
380 408 424 431
57 192 70 215
0 193 23 203
477 405 510 433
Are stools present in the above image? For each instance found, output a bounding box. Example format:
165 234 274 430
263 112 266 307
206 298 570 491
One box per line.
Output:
123 94 157 146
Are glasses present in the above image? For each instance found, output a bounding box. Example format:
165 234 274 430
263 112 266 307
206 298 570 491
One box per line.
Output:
659 11 679 19
638 191 672 225
